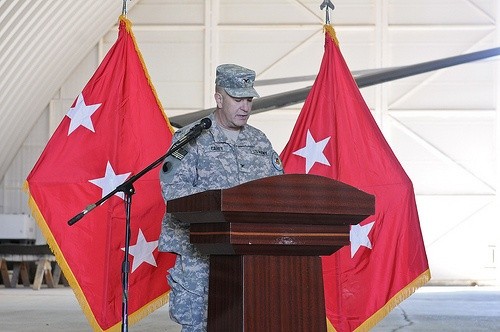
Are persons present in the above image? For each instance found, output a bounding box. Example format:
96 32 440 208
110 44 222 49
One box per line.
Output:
158 64 284 332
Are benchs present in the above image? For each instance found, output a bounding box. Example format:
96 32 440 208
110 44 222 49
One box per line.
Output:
0 238 67 290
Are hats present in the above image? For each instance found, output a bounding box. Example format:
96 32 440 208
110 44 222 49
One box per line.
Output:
215 63 260 99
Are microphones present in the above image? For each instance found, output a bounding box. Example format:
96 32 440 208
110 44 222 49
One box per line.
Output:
174 118 212 145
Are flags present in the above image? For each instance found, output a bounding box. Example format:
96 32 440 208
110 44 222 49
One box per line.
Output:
24 13 176 332
279 23 431 332
